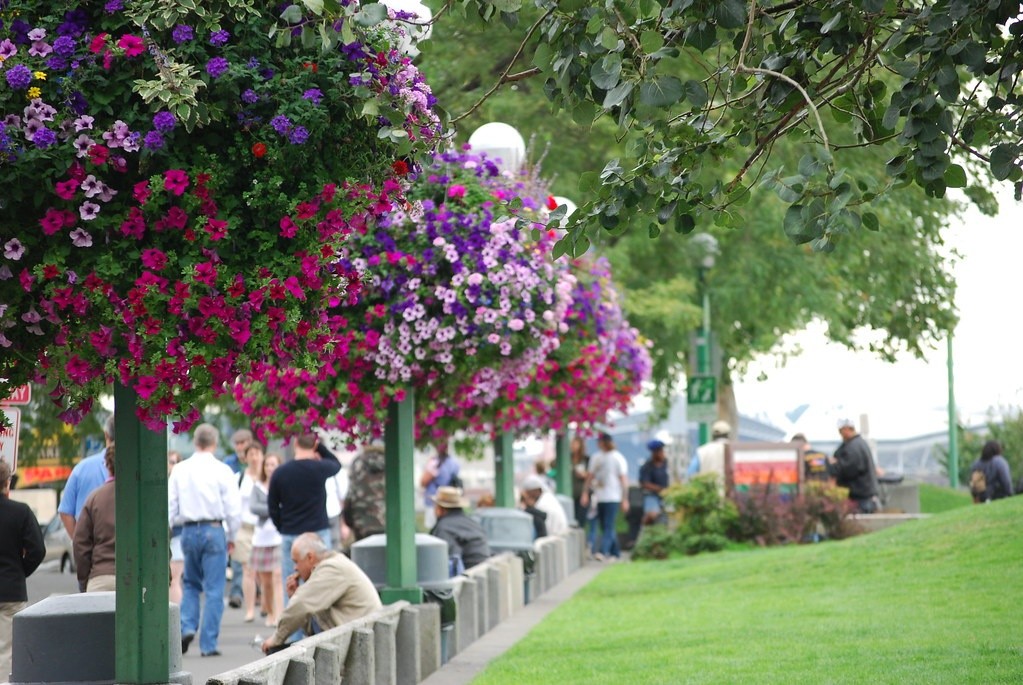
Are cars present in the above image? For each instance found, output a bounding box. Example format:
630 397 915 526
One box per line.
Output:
37 511 76 572
469 511 533 552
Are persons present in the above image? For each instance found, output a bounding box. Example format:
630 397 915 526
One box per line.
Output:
684 420 734 499
570 432 629 560
58 414 116 594
419 443 460 532
0 462 46 684
789 433 839 538
429 457 567 575
635 439 670 545
168 423 385 656
828 418 880 514
967 440 1014 504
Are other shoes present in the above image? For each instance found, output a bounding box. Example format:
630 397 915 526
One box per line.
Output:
594 552 606 561
201 651 219 656
182 634 194 653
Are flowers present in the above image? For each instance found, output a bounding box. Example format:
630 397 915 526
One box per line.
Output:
0 1 654 459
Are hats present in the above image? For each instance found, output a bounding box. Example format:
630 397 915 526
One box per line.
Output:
712 422 730 436
429 485 468 509
838 418 855 431
648 440 665 449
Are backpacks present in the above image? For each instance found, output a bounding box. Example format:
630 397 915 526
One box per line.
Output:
970 459 987 492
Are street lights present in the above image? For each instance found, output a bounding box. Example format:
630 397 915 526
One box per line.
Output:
683 230 721 445
461 121 527 507
359 0 433 604
531 194 579 498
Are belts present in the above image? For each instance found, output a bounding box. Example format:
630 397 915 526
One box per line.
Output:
185 520 221 526
